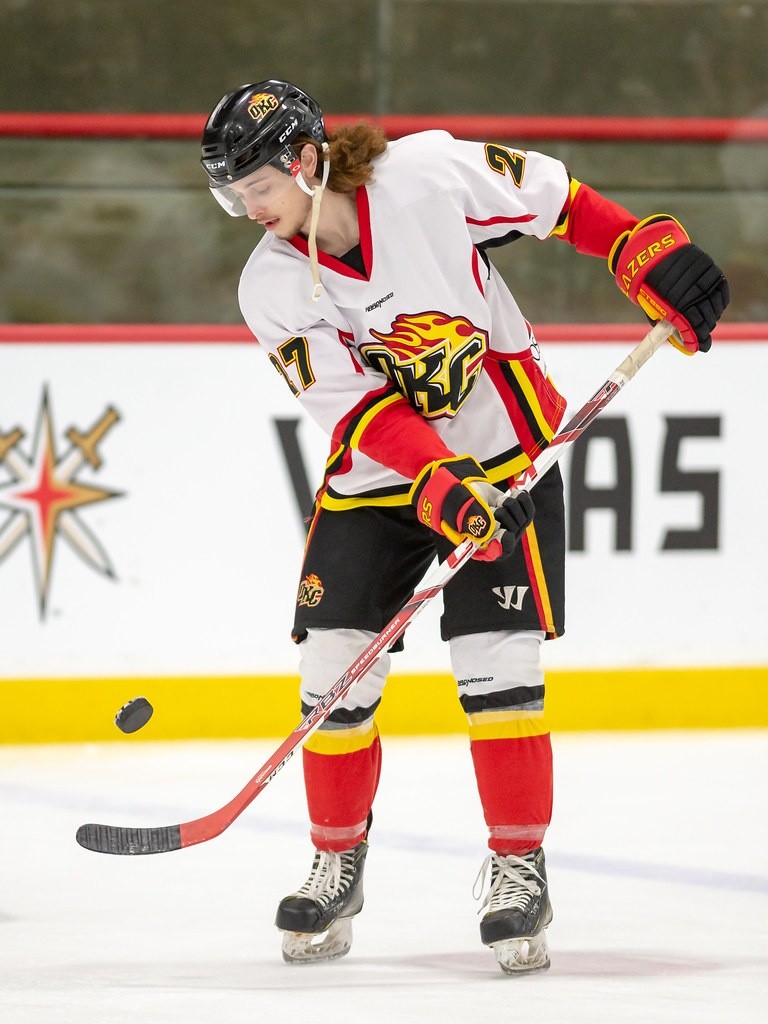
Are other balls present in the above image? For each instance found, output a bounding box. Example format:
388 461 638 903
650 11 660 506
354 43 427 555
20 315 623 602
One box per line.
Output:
115 698 151 734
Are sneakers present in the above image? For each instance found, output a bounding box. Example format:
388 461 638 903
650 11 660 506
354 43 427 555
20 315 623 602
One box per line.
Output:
472 853 553 972
273 837 367 961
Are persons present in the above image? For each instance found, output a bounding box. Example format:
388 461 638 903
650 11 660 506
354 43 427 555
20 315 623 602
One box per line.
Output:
197 78 730 976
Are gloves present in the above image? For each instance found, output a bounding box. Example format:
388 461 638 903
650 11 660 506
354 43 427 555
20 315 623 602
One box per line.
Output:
604 211 728 357
407 453 534 559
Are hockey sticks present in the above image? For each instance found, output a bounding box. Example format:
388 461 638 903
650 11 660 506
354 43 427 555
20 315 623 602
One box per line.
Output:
76 318 674 855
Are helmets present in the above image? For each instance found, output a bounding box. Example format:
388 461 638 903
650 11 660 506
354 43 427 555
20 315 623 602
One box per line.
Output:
202 77 325 187
